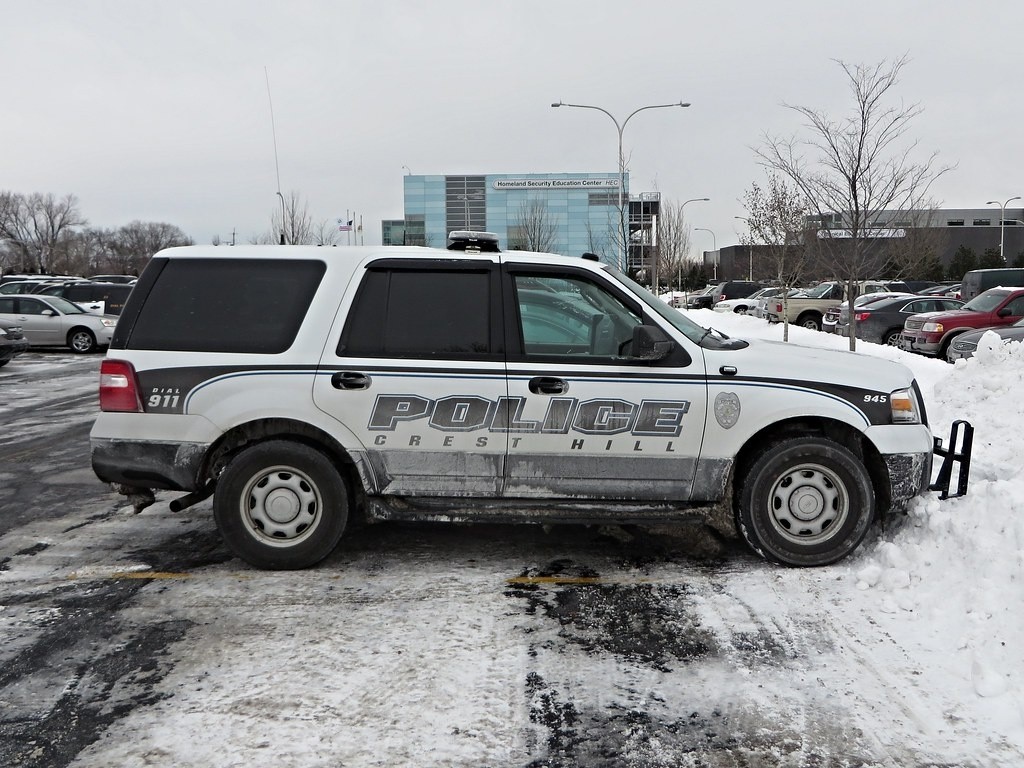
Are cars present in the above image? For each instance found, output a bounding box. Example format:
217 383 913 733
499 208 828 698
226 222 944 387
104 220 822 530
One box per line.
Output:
673 267 1024 333
0 294 121 354
0 275 138 366
835 296 978 351
947 316 1024 364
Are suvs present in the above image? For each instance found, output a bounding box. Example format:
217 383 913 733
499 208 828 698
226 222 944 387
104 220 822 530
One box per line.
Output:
901 285 1024 362
90 231 975 569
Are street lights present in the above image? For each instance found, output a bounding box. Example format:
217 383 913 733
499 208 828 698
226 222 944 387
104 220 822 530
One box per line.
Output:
678 198 711 291
552 102 691 276
277 192 286 240
694 228 717 279
986 197 1021 256
735 216 752 282
